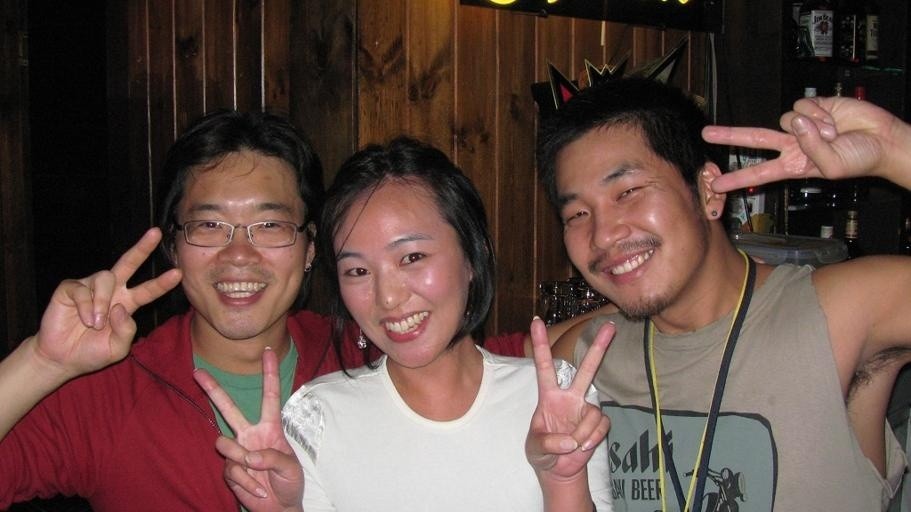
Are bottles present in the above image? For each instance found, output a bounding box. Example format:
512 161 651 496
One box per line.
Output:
539 276 609 327
781 0 885 261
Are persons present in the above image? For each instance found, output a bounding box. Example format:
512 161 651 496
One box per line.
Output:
0 105 766 512
190 135 619 512
536 75 911 512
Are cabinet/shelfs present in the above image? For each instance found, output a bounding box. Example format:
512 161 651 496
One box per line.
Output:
769 0 911 246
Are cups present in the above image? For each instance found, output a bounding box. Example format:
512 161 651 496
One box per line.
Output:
751 214 772 234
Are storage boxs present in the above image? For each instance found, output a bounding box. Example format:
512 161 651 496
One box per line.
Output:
730 230 848 270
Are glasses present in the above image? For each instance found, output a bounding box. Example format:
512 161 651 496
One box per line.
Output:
178 220 308 249
